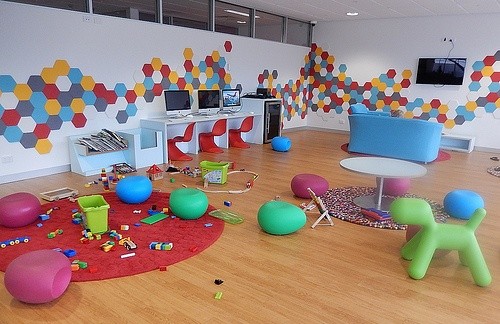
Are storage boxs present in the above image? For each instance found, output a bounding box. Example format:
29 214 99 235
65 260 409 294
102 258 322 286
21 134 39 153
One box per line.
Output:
199 161 229 185
77 195 110 233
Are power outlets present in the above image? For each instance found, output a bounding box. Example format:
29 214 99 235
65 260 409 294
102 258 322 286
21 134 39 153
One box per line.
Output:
441 36 456 44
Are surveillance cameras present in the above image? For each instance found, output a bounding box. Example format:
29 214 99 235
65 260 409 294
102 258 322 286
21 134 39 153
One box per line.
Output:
311 21 317 27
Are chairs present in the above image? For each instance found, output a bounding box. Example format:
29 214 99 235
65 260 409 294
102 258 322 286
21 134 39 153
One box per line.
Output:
229 116 254 148
167 122 196 161
199 118 227 153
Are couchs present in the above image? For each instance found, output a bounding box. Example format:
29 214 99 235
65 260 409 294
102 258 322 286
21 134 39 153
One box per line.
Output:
348 103 444 164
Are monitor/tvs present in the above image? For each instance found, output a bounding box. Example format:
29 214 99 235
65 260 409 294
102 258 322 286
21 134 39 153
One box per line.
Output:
197 90 221 113
223 89 241 110
415 57 467 85
163 90 192 114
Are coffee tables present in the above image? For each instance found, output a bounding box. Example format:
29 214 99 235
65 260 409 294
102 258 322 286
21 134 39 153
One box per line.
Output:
440 134 475 153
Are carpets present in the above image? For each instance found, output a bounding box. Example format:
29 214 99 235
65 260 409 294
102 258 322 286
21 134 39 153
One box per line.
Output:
342 143 451 162
321 186 451 230
0 193 225 282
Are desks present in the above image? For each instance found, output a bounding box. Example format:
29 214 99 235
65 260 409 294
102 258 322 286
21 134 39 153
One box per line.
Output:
140 111 261 164
341 155 427 212
